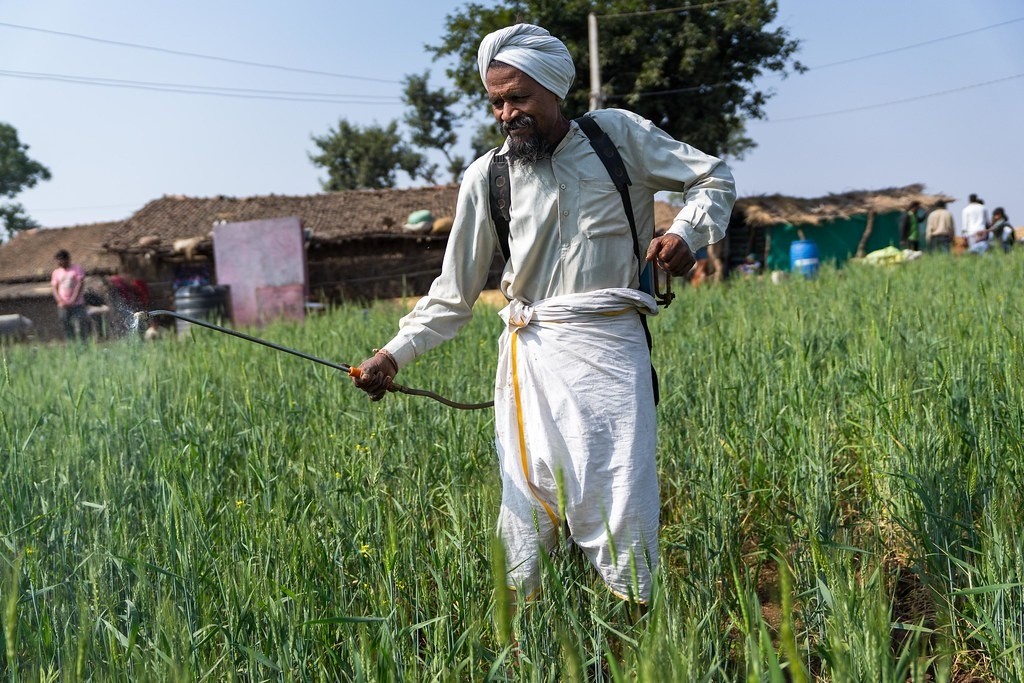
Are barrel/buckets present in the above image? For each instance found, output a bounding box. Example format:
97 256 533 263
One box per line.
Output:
212 285 233 327
789 239 820 280
173 285 215 342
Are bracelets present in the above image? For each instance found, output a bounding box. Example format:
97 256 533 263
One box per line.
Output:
373 349 399 374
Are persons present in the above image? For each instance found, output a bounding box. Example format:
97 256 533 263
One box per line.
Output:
976 208 1015 253
926 200 954 257
51 251 87 342
737 254 761 276
900 200 932 251
352 24 736 683
961 194 991 252
